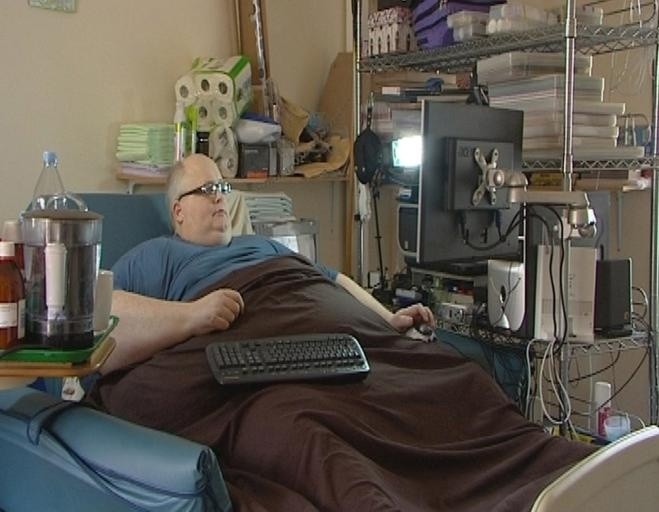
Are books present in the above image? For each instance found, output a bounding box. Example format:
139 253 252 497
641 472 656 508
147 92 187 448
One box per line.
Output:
360 79 488 143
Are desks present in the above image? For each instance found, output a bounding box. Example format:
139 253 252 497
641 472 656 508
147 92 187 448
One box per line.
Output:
0 332 116 379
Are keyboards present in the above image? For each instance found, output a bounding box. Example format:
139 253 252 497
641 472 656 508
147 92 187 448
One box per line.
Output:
205 332 370 385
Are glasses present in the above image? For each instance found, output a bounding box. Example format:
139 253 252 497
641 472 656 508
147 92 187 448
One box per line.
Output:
177 179 234 201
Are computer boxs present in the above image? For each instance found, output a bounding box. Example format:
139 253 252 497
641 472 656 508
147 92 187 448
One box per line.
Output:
527 245 593 344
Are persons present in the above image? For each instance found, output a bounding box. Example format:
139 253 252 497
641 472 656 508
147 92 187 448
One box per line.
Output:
87 153 604 512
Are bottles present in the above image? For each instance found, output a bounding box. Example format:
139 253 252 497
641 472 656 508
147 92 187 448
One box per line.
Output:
595 381 611 436
0 217 29 342
0 241 25 347
31 146 66 209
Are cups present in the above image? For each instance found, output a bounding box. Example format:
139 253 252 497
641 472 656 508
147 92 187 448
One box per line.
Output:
604 416 630 439
91 265 116 333
22 207 104 351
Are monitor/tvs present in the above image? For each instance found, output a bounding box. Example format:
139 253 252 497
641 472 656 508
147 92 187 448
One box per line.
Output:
418 98 524 264
396 203 418 256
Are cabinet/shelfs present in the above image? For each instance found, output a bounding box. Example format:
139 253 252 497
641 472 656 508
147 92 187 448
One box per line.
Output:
350 0 658 448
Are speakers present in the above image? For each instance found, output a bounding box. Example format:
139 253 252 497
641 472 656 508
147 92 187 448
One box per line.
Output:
486 257 530 340
596 257 633 336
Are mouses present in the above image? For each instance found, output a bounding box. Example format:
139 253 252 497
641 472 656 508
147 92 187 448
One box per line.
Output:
403 324 437 342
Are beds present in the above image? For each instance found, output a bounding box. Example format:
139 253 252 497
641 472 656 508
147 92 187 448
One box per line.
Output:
1 192 659 511
